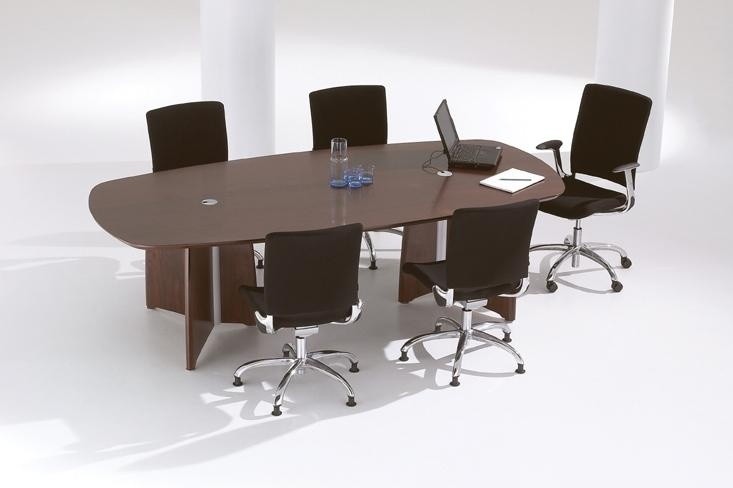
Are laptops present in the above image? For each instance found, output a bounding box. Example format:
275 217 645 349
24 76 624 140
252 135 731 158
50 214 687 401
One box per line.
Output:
433 98 503 170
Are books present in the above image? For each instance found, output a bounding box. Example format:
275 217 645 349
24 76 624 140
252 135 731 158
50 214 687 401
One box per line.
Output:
479 168 545 194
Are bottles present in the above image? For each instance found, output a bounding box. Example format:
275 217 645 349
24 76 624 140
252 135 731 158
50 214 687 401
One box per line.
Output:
348 163 374 188
328 137 349 188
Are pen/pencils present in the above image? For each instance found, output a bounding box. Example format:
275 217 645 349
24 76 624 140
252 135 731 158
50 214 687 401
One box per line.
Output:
500 179 532 181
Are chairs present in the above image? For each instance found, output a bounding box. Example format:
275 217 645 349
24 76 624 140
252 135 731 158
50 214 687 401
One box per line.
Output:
310 85 388 149
537 83 652 292
233 223 364 417
146 101 229 171
399 199 539 386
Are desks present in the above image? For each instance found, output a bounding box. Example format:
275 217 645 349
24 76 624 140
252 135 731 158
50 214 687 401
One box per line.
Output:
88 137 564 370
